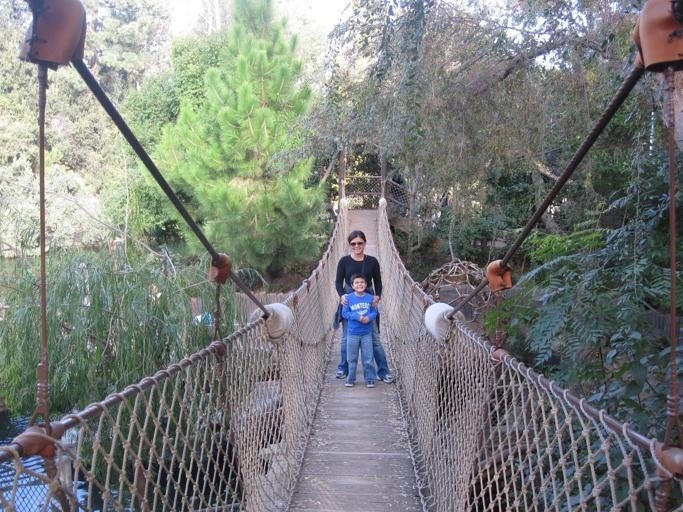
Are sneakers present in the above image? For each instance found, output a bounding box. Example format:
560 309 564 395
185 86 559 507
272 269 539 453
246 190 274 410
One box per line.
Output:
334 369 396 388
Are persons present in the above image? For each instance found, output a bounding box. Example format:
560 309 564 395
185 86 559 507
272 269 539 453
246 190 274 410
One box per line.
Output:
390 173 451 218
341 273 380 388
332 230 394 384
